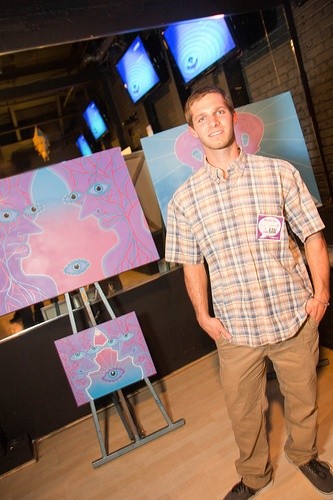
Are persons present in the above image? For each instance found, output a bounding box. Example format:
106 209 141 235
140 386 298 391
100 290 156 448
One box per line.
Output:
164 85 333 500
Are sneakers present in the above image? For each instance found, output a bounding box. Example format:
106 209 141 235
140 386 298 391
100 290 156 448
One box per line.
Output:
299 458 333 500
225 474 272 500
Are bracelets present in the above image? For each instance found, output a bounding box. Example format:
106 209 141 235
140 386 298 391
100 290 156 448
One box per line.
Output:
313 297 329 306
